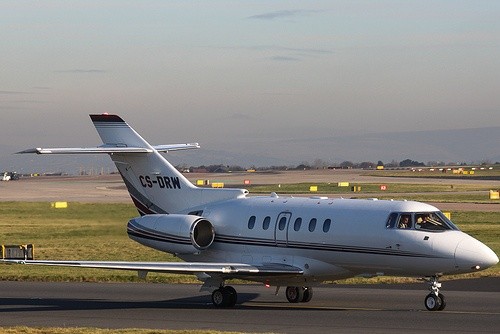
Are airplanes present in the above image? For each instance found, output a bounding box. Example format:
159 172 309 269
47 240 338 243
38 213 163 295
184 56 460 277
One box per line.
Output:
2 113 500 311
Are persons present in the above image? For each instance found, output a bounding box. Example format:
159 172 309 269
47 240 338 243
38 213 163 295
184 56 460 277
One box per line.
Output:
398 216 409 228
421 215 439 227
